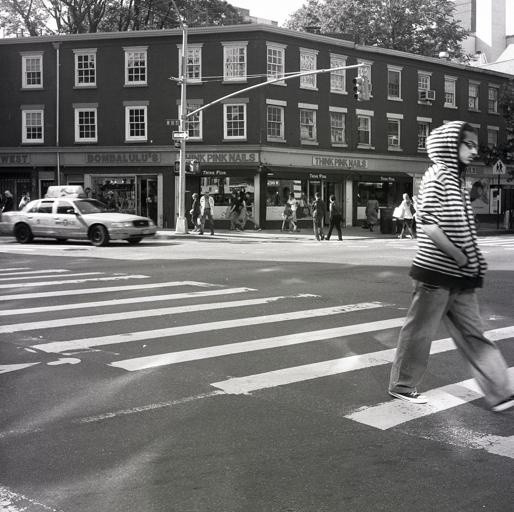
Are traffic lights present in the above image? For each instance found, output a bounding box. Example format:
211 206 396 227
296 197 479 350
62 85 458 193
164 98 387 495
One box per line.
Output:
174 160 181 172
365 79 373 102
351 77 364 101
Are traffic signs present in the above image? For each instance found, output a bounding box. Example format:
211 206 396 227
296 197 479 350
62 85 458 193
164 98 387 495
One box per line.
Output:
171 131 188 140
163 119 179 126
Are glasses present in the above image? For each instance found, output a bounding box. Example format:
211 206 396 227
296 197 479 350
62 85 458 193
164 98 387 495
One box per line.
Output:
460 139 477 149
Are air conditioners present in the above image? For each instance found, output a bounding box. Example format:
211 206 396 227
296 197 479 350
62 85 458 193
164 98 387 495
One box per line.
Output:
389 137 398 146
419 90 436 102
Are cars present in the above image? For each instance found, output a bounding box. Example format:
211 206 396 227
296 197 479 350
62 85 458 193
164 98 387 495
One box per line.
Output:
0 185 158 247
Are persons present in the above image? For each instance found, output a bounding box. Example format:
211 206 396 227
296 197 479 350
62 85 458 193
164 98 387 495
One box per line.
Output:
198 191 215 235
84 187 116 210
228 189 261 233
365 193 380 232
324 195 343 240
387 117 514 412
397 192 418 240
190 192 201 232
311 192 326 241
280 191 299 234
0 190 30 212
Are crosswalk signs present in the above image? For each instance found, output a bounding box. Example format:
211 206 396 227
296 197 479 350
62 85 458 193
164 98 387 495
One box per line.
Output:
492 159 507 175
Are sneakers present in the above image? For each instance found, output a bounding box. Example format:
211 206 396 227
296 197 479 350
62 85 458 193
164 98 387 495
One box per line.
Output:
490 393 514 412
388 386 428 404
186 223 416 244
